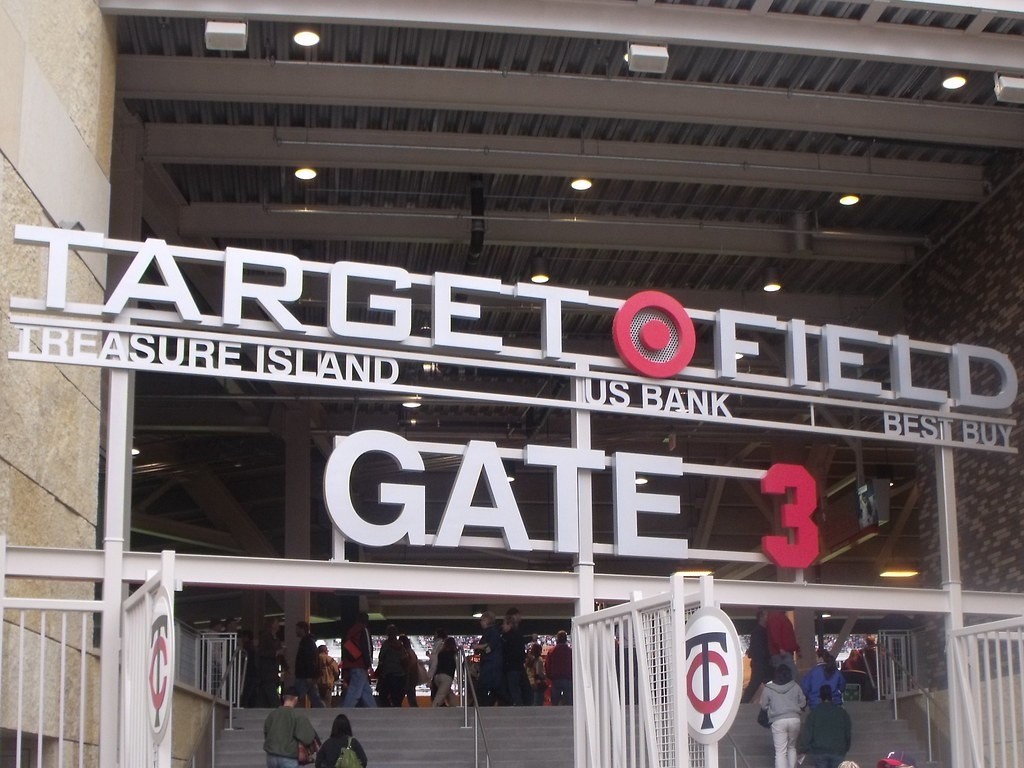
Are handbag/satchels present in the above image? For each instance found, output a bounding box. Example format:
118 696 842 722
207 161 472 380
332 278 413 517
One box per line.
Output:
534 675 550 689
335 737 363 768
417 660 429 686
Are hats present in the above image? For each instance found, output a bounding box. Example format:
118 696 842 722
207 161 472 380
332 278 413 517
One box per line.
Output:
851 649 862 655
877 751 917 768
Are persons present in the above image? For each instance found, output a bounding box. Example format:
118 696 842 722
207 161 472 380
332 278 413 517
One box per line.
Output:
263 686 314 768
205 607 572 707
315 714 368 768
741 607 915 768
